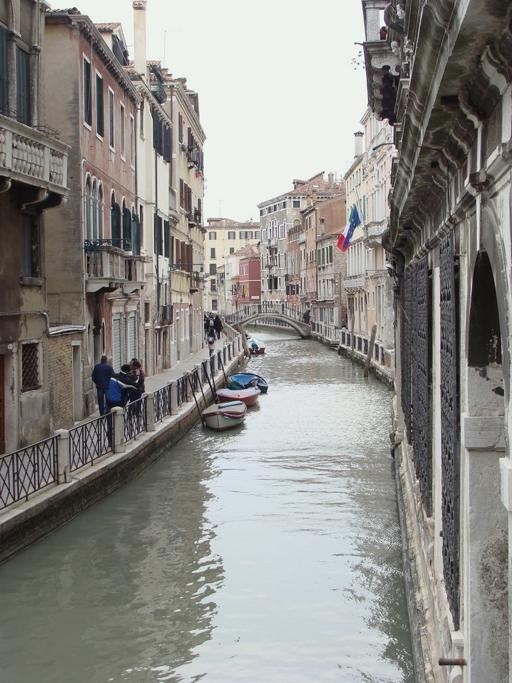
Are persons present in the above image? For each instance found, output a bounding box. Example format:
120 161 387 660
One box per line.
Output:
303 309 311 324
104 357 145 447
90 353 114 417
204 310 223 357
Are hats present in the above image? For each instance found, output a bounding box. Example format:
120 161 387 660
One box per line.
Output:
120 363 131 372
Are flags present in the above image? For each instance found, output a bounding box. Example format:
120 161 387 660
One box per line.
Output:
342 206 361 249
336 206 354 251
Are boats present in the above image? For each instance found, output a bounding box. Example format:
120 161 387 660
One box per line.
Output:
201 373 268 428
247 338 266 354
